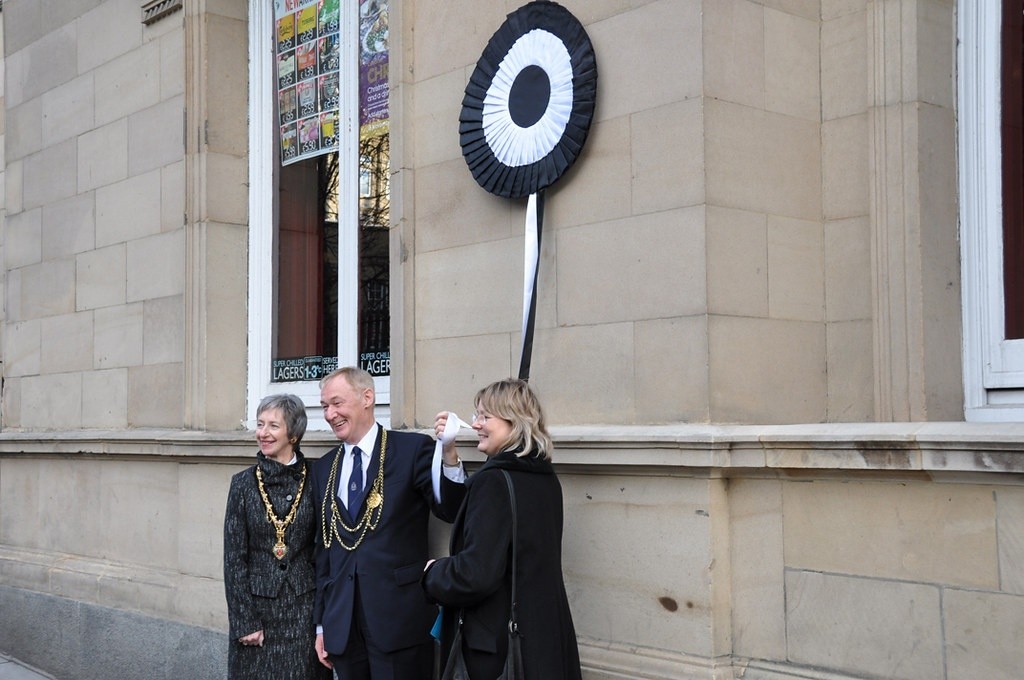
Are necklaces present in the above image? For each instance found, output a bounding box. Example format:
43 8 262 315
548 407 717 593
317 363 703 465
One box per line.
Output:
322 427 387 550
256 463 306 560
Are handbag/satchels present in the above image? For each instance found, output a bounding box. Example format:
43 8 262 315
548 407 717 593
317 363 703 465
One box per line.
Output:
442 619 524 680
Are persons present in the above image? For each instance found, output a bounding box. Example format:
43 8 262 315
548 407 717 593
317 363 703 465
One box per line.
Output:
310 366 469 680
420 379 582 680
224 394 333 680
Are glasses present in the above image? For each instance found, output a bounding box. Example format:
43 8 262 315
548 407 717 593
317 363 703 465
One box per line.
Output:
472 412 507 425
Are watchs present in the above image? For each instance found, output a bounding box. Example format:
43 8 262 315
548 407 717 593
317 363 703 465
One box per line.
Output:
443 456 460 467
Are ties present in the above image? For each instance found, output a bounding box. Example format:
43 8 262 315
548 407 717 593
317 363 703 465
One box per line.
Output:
347 445 362 511
